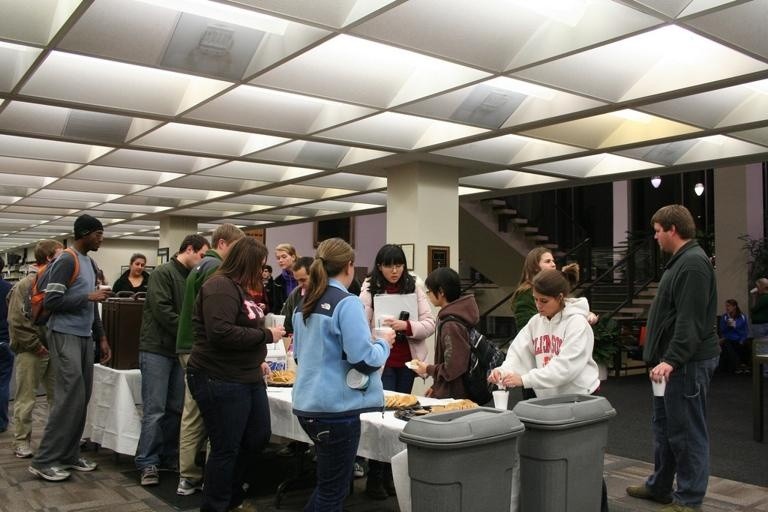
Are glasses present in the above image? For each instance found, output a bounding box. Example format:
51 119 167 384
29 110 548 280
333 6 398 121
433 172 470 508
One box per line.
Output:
426 287 436 297
379 264 404 272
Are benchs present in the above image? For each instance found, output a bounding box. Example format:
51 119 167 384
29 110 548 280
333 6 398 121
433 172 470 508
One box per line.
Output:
609 314 755 380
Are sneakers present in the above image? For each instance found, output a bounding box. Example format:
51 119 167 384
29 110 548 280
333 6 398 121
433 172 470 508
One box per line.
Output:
354 463 364 477
176 478 205 496
140 464 159 485
626 480 673 504
13 440 97 481
280 442 310 456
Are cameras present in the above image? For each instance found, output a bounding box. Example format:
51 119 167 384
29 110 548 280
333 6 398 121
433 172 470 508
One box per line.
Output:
394 311 410 342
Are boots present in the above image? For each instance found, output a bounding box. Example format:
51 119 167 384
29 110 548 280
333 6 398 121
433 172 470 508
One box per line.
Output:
366 460 389 498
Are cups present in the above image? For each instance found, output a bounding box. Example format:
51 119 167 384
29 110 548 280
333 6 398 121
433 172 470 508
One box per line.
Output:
272 314 286 329
374 328 392 346
98 284 110 302
648 373 666 398
493 389 511 411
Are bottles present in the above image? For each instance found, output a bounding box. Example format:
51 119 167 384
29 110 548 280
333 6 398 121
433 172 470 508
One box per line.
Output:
396 311 410 343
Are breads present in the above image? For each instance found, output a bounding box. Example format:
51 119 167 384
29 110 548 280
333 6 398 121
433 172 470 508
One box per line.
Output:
411 359 420 367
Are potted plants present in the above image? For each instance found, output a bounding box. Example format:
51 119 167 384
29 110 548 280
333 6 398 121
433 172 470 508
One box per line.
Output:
589 320 624 382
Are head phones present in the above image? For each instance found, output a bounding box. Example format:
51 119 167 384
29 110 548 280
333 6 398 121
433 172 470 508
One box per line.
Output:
77 218 90 237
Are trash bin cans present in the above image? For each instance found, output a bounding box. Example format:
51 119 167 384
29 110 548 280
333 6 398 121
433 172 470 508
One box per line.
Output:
511 393 617 512
399 407 527 512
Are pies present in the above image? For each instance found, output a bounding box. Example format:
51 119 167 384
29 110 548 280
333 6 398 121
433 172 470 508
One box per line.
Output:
414 397 479 416
265 369 294 385
382 394 420 410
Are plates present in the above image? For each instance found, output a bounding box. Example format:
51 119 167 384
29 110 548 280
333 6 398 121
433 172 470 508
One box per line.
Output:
395 406 434 421
266 381 293 388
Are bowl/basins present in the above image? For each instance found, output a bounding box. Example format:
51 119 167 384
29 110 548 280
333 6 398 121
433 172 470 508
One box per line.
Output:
405 361 425 370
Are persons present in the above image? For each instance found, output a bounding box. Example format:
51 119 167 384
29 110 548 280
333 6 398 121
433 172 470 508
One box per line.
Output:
358 244 436 394
750 277 768 378
719 298 753 376
260 244 314 337
414 267 480 401
624 203 723 512
176 224 244 495
0 215 112 480
510 247 599 400
134 234 211 485
112 254 150 294
186 236 286 512
487 263 609 512
291 237 396 512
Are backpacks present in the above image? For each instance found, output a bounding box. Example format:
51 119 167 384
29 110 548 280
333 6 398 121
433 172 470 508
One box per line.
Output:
435 315 506 405
31 247 78 325
